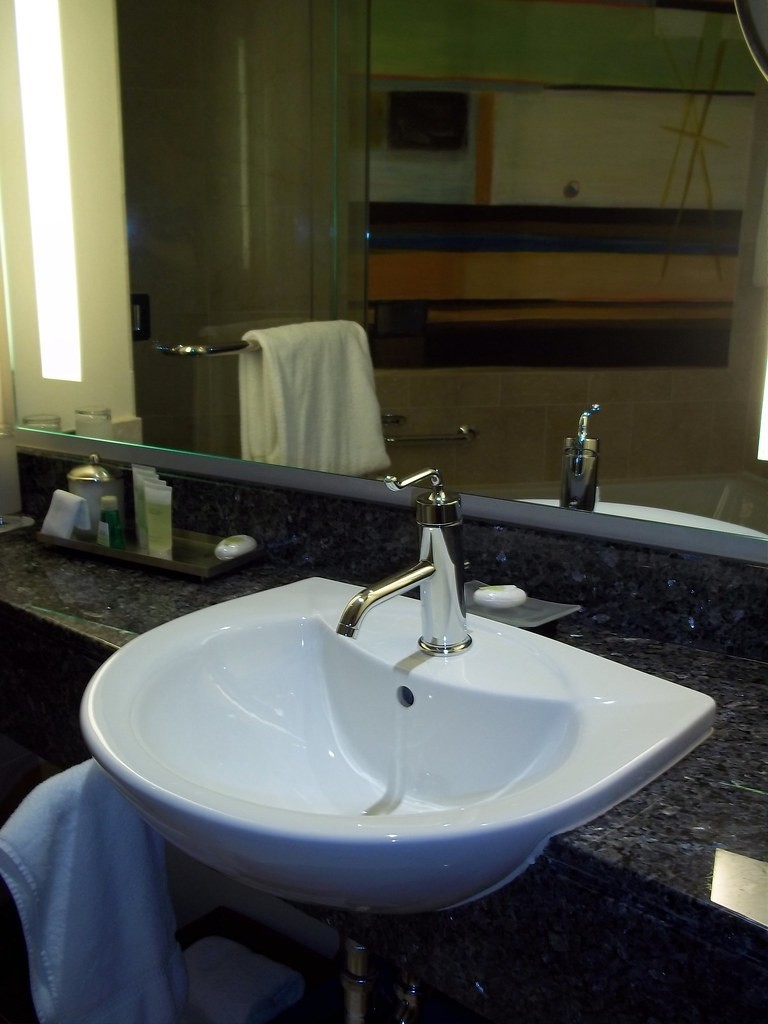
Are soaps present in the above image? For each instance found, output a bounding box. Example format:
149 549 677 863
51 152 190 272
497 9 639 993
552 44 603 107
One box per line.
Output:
215 534 258 560
474 585 527 609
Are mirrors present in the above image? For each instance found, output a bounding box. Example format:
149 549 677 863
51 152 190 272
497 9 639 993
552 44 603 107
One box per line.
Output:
0 0 768 541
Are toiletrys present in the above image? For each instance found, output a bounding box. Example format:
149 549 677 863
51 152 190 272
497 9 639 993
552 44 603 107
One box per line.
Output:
144 481 173 560
96 495 128 550
132 463 165 553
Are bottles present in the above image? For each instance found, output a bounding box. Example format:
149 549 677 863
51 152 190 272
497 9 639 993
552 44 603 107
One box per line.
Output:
23 415 61 432
73 406 112 438
96 495 126 550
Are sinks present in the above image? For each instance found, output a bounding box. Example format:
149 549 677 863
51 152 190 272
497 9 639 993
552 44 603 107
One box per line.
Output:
79 574 718 915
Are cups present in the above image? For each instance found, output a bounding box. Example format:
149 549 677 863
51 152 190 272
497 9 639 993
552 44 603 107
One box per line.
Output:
65 453 127 542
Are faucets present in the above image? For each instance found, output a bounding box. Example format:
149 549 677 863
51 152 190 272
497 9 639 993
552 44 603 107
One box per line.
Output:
337 467 472 658
559 402 603 512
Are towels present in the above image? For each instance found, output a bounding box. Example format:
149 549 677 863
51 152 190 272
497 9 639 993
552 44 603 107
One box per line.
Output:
0 760 191 1024
179 933 306 1024
237 319 393 477
